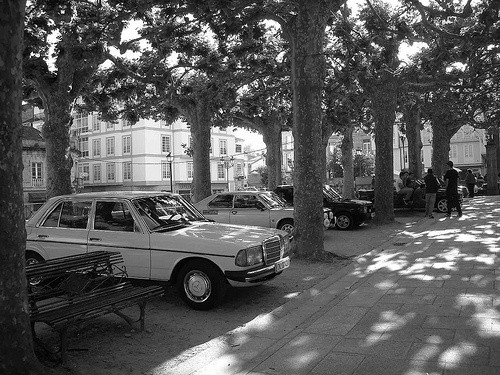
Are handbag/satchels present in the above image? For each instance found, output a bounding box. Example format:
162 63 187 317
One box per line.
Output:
474 185 478 193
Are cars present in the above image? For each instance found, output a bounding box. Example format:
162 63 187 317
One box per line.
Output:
193 191 336 240
25 190 295 310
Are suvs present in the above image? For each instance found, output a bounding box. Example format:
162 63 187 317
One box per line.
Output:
274 184 374 230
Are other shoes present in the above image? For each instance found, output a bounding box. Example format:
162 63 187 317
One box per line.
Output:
457 212 462 217
424 214 429 217
445 213 451 216
430 215 434 218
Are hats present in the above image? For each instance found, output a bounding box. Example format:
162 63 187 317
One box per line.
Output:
427 168 435 173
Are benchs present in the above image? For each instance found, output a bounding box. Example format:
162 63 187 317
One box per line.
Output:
26 252 167 371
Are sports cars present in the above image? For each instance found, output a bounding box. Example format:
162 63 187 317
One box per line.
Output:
358 179 464 214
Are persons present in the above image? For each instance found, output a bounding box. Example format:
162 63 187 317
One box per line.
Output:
443 161 462 217
396 171 419 205
424 168 440 219
465 168 488 198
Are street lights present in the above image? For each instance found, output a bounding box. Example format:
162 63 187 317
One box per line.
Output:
165 152 175 192
220 155 236 191
